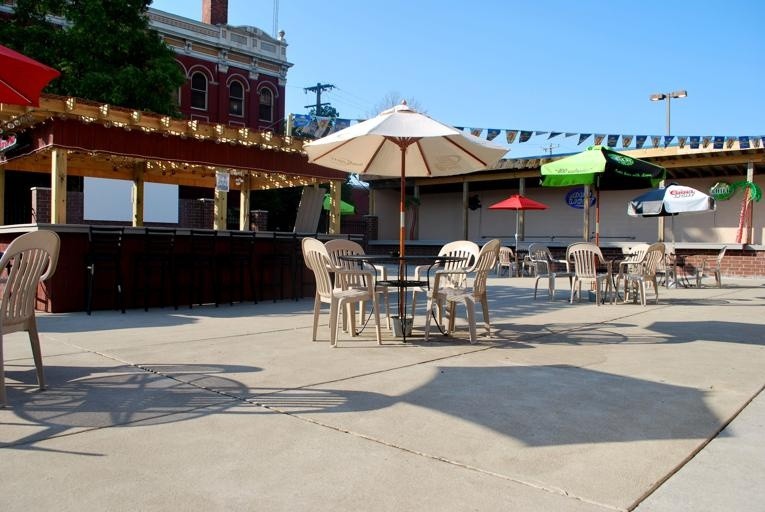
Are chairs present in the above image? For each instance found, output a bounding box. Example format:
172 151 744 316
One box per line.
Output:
0 232 61 408
81 226 369 315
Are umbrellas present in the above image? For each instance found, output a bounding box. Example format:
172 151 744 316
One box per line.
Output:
302 99 511 316
488 193 552 263
625 181 717 280
0 44 63 110
540 142 668 289
324 193 358 217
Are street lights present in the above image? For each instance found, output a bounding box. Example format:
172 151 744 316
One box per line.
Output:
648 90 688 137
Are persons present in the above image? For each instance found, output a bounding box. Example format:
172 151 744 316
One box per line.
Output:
250 213 260 231
